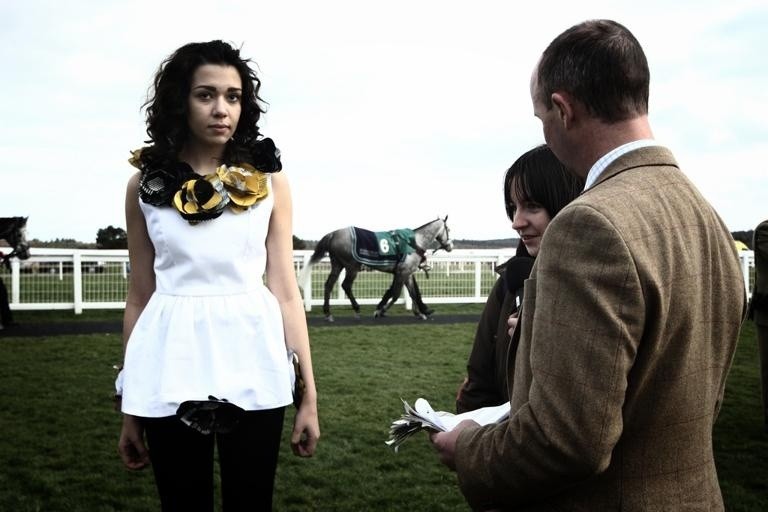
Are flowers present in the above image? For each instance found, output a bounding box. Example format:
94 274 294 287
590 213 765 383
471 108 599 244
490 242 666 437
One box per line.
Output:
129 145 268 223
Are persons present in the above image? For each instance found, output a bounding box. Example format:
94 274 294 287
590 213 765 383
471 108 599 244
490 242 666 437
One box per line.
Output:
451 143 589 417
427 18 750 511
114 37 322 510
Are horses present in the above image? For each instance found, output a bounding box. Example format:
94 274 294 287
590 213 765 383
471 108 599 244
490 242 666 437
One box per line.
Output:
0 215 31 261
296 213 455 324
